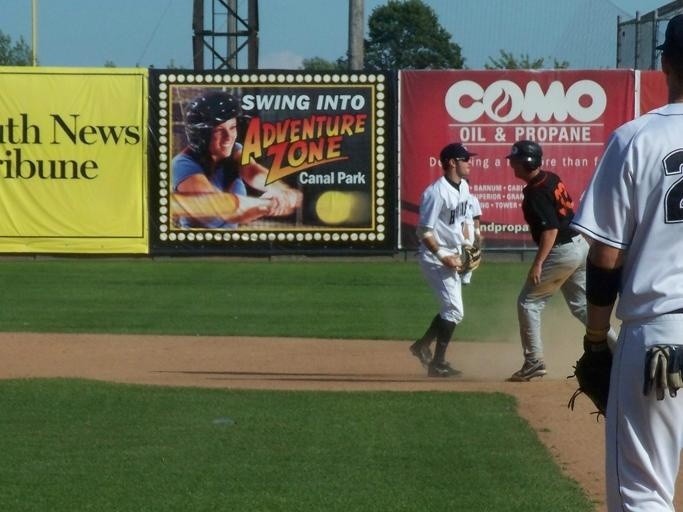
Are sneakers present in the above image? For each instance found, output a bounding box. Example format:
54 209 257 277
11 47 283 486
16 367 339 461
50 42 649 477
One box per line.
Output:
512 359 547 381
428 362 462 377
410 340 432 369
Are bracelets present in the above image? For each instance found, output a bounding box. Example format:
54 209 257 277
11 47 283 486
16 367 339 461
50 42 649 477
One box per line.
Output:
474 228 480 235
434 250 447 259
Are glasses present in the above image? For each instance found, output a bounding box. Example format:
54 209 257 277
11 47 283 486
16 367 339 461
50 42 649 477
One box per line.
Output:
454 156 469 162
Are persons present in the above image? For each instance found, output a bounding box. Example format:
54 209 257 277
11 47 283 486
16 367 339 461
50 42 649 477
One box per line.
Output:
570 13 683 511
502 141 616 382
409 143 481 378
171 93 295 230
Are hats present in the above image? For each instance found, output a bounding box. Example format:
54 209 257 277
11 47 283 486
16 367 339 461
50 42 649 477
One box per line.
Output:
655 13 683 49
441 143 478 162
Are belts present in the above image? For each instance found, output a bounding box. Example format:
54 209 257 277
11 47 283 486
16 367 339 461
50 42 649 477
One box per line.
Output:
557 239 573 244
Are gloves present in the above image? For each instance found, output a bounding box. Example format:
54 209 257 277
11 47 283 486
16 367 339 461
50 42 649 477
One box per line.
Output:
643 345 683 400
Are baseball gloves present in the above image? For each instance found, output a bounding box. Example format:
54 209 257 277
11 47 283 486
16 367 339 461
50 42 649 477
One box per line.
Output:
568 348 613 420
457 245 481 276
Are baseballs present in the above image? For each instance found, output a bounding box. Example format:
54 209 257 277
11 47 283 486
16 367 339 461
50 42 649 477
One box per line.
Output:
316 191 353 224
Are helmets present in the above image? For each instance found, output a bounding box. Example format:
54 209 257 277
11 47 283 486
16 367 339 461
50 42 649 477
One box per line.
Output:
505 140 542 170
184 94 242 155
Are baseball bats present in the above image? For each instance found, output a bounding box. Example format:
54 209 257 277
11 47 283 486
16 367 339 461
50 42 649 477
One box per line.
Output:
173 193 272 218
